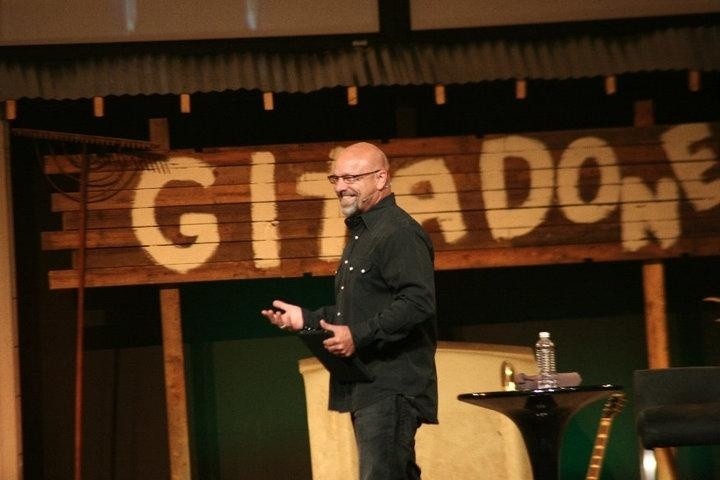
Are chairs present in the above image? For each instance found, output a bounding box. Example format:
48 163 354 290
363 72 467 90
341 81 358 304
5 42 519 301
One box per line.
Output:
631 366 720 479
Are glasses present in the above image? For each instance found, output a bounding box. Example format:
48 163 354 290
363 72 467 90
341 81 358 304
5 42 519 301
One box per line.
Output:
328 170 380 185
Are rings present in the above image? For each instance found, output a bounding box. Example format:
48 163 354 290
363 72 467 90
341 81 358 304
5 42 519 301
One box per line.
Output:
278 325 287 330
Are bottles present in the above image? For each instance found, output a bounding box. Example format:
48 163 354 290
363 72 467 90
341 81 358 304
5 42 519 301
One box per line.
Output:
534 331 558 390
501 360 516 392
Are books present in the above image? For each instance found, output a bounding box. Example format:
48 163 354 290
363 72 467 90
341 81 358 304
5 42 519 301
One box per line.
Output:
297 331 374 384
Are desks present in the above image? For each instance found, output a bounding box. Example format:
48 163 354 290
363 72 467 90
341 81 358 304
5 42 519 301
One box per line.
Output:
457 384 624 479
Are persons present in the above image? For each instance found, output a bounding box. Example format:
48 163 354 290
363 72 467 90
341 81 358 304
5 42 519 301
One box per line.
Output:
260 142 439 480
